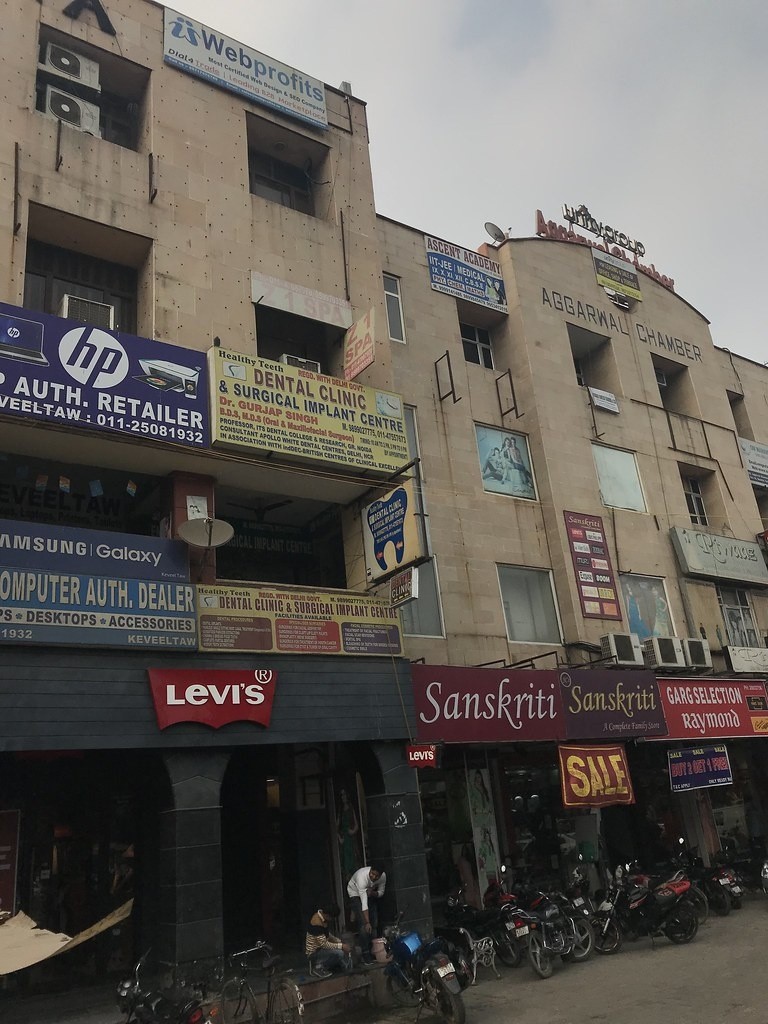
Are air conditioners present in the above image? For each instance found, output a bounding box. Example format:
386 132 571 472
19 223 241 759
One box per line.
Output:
55 294 115 330
274 354 321 375
654 369 667 387
42 84 100 139
680 638 713 672
642 636 686 670
45 42 99 88
599 632 644 668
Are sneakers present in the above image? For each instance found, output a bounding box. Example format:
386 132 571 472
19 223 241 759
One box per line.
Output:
360 951 377 966
309 960 333 978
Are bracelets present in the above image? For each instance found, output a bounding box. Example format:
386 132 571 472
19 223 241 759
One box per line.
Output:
365 922 371 925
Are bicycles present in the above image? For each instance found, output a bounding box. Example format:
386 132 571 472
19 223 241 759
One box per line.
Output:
220 940 303 1024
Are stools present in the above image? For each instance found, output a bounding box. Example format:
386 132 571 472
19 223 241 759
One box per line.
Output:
509 468 527 487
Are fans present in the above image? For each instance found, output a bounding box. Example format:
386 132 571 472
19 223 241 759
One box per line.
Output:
225 497 295 523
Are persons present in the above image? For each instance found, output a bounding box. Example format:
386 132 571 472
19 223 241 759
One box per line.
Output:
306 905 354 977
347 862 387 965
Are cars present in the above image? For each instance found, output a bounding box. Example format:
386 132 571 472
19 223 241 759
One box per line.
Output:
516 832 575 856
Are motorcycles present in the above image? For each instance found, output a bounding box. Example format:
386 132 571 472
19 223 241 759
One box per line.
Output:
443 837 744 979
118 948 219 1024
384 903 473 1024
